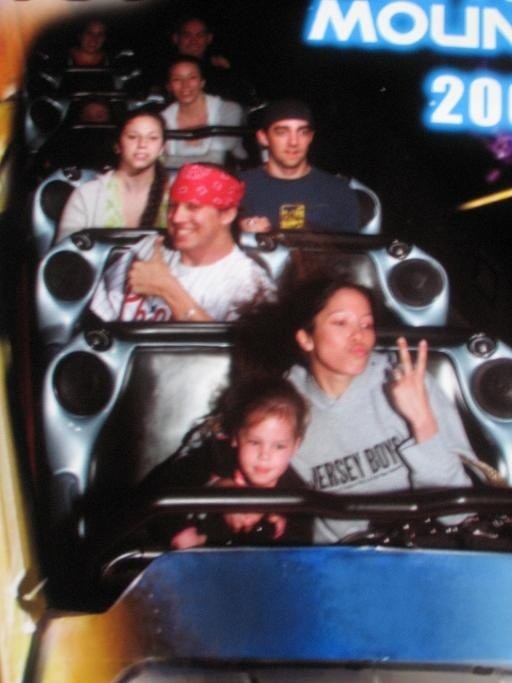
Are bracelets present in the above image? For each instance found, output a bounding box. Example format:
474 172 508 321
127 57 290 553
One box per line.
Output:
245 217 256 226
177 301 198 320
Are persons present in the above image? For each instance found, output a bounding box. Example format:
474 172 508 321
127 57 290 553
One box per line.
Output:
66 17 109 67
52 104 166 246
225 93 364 234
79 98 116 123
84 159 277 324
152 53 252 181
267 273 471 545
107 377 322 550
167 10 232 68
